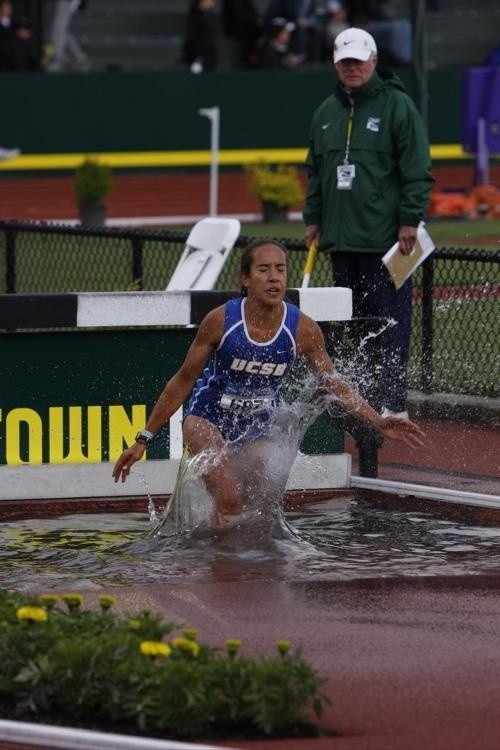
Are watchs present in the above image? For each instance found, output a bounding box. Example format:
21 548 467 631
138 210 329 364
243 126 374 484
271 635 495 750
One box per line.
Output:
134 431 152 445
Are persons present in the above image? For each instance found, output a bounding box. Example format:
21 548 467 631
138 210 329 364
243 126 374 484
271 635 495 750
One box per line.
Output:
110 238 429 539
0 1 86 74
181 0 408 75
300 27 436 421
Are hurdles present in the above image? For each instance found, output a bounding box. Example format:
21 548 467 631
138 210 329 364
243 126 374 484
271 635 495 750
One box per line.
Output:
0 286 358 503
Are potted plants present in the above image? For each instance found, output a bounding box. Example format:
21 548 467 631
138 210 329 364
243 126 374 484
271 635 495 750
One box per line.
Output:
73 157 114 226
236 156 306 224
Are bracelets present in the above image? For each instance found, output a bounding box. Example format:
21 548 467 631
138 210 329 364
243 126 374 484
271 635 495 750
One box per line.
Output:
141 428 154 439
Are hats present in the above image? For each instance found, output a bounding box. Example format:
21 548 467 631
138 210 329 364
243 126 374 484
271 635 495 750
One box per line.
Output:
333 27 377 65
